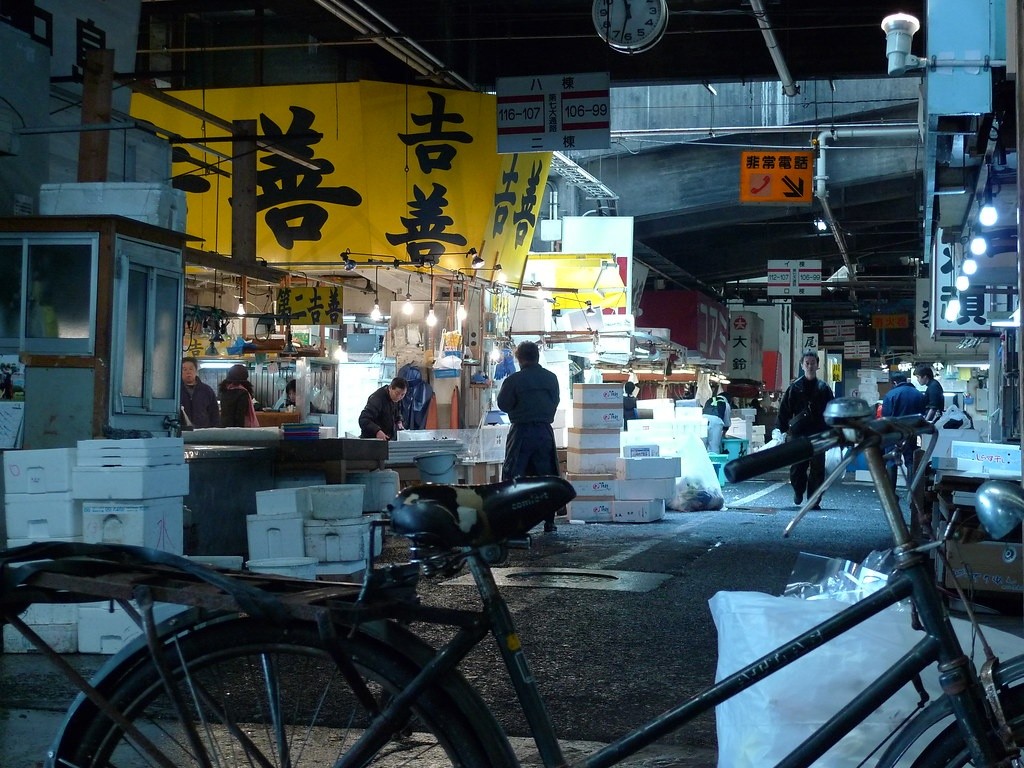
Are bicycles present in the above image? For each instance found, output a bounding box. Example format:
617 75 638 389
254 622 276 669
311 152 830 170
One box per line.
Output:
0 396 1024 768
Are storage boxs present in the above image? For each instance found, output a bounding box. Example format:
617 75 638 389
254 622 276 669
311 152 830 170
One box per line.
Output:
859 377 879 407
2 439 383 654
729 408 766 452
540 349 682 522
37 182 187 234
921 429 1021 507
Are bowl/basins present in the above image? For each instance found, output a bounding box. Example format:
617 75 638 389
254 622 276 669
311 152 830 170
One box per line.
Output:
309 483 365 519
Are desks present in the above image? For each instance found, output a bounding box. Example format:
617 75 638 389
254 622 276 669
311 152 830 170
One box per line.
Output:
187 438 388 484
385 461 504 485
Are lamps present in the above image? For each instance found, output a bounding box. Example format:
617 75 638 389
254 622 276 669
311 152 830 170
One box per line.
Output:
881 13 1006 76
339 248 397 272
282 319 298 355
421 247 485 269
458 264 507 283
506 281 544 299
814 219 827 229
205 327 225 356
554 297 596 316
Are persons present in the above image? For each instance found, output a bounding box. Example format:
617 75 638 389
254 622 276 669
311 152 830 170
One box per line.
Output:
777 351 835 511
181 356 220 428
703 392 732 435
286 379 315 413
0 363 12 399
880 371 926 495
496 341 560 533
913 364 945 423
220 364 260 428
359 377 409 441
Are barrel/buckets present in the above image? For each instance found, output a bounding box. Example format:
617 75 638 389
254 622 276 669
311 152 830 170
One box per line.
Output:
413 450 461 483
245 557 319 581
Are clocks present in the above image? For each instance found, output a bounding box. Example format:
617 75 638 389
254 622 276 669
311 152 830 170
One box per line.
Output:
592 0 669 56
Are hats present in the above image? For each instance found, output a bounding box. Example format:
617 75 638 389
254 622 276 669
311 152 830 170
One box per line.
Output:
228 364 249 382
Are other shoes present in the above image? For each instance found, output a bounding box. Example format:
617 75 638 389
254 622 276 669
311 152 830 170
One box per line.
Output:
544 523 557 531
810 504 821 510
794 484 806 504
